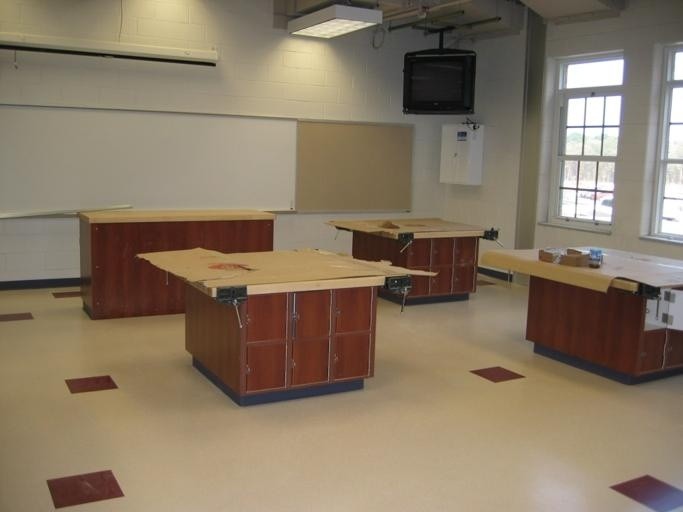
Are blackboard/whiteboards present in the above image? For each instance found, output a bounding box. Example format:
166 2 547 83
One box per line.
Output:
1 101 298 219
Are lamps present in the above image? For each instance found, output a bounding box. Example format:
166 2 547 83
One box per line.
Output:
287 4 384 41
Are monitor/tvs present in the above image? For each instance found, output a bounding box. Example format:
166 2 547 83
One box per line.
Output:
402 49 477 115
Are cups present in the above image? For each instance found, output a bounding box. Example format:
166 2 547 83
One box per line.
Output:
589 247 603 269
